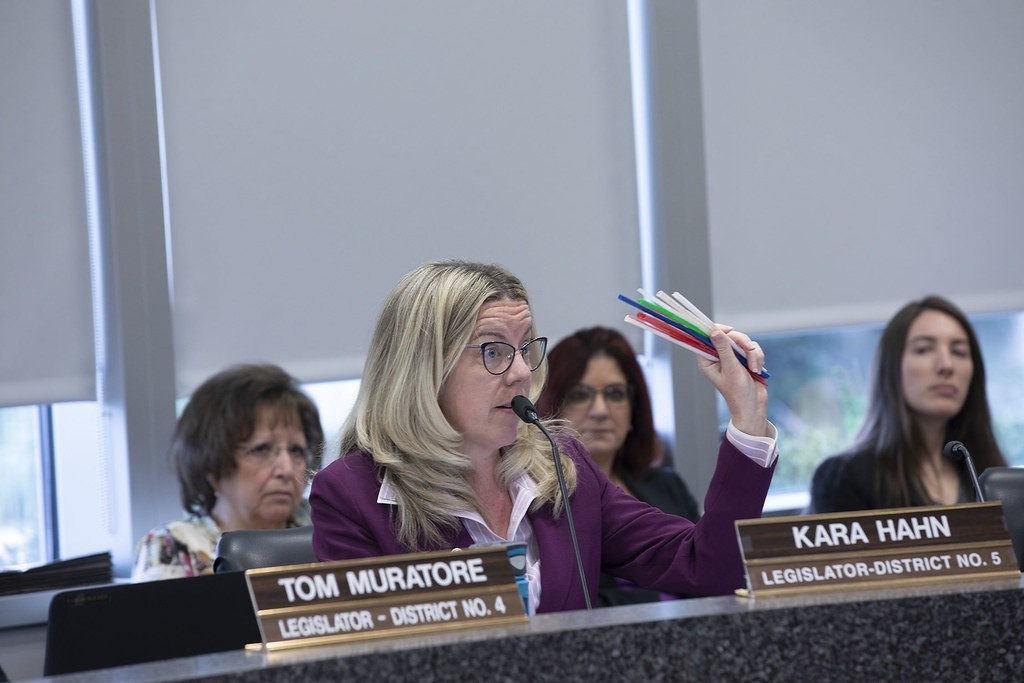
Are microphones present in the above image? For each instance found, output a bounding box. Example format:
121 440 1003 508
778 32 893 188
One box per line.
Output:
509 394 592 609
943 440 983 502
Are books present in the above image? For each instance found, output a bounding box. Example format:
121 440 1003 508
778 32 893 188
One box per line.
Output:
0 551 112 596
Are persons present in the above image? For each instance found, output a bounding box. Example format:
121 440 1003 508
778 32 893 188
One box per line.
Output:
130 365 325 586
533 323 700 611
804 295 1009 514
310 259 781 615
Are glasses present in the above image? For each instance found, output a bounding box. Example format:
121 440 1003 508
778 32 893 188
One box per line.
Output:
565 383 629 405
448 336 548 376
